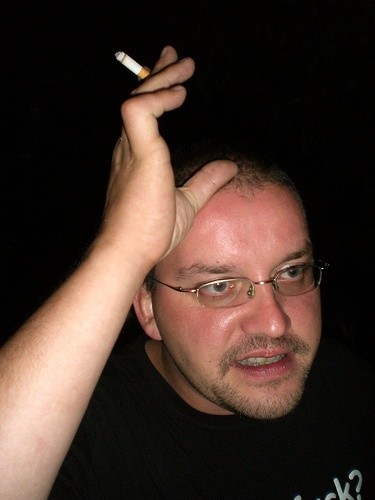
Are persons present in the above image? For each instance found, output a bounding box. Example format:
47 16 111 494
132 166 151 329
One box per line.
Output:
0 45 375 499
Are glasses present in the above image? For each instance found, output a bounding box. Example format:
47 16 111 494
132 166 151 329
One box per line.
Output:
152 258 330 309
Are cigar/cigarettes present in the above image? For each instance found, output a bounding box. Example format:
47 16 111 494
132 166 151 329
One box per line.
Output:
114 51 150 78
